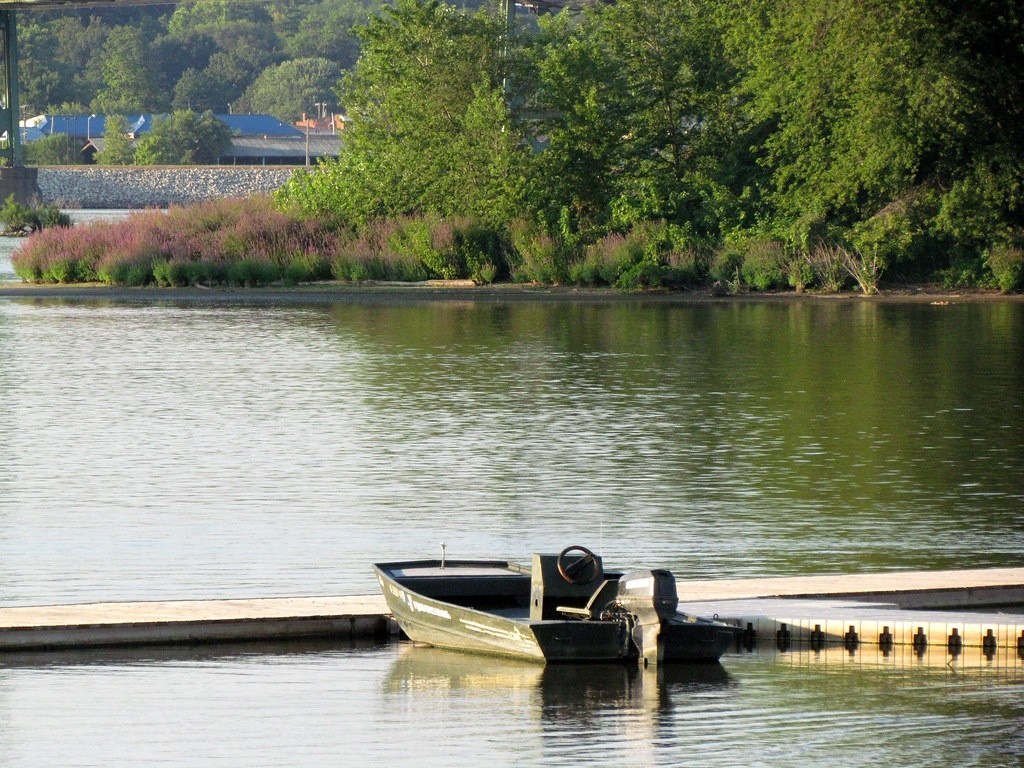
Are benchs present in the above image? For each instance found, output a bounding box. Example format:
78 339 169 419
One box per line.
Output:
386 566 533 606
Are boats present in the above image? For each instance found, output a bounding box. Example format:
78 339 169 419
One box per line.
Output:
371 546 741 665
